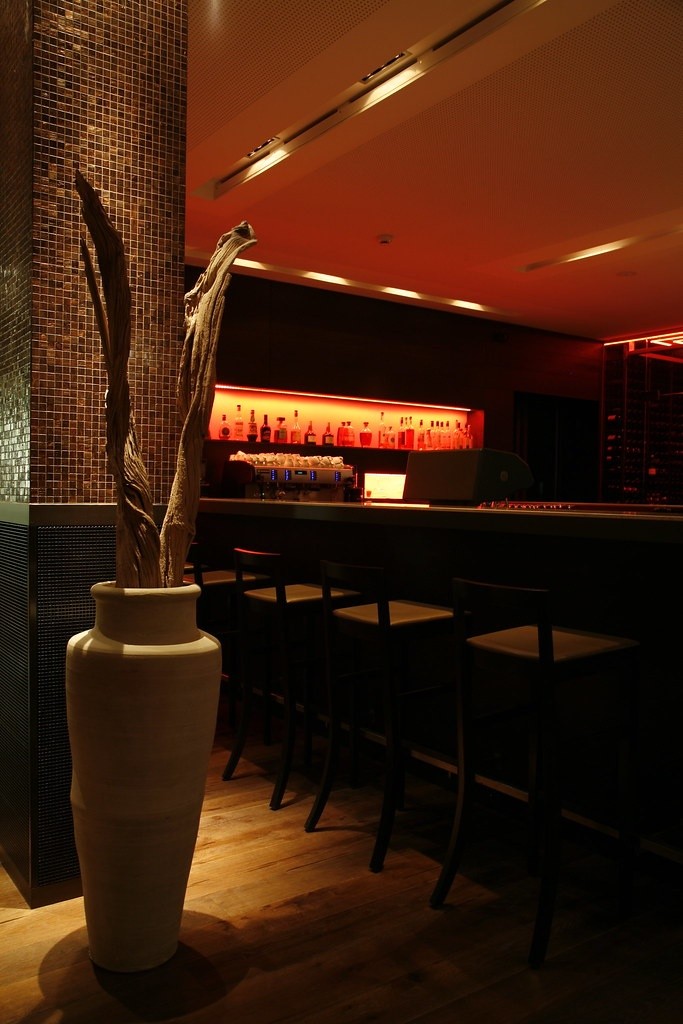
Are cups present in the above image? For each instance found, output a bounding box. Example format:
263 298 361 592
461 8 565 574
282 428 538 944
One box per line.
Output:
230 451 344 469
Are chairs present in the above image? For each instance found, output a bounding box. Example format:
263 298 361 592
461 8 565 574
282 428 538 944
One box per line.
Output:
222 547 362 810
304 559 469 873
184 542 271 730
431 577 639 969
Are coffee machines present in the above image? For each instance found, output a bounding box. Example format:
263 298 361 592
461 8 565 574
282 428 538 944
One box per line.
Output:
224 461 362 503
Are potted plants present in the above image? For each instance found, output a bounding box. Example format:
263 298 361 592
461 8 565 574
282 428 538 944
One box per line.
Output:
65 159 258 973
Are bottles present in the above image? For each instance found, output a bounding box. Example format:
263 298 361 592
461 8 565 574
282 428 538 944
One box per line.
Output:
219 415 232 440
233 405 244 441
247 410 258 441
304 421 317 444
337 422 355 446
274 418 288 442
322 422 335 447
290 410 301 443
260 415 271 442
377 412 395 449
360 422 372 447
398 417 414 449
418 419 474 449
